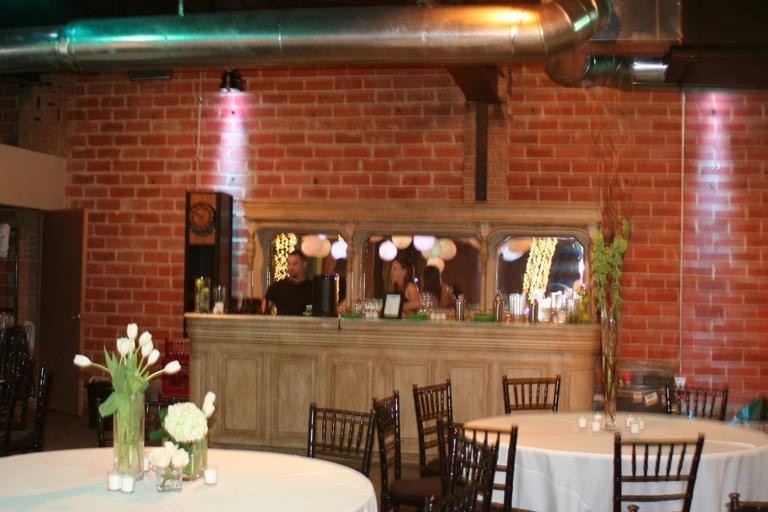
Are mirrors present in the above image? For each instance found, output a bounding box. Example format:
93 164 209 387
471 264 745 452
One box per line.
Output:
250 222 599 323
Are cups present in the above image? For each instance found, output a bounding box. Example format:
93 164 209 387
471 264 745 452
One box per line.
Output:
625 416 633 426
637 420 644 428
204 465 217 483
213 286 226 313
594 413 601 420
121 473 137 494
107 471 120 491
632 421 637 432
576 417 586 429
592 418 600 431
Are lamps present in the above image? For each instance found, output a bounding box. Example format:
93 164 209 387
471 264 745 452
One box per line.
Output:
218 69 246 95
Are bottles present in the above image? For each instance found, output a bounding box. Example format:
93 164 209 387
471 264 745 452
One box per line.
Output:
354 297 382 319
494 294 503 321
528 299 538 322
455 294 465 320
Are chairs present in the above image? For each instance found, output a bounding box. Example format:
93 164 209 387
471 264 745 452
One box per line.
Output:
666 382 730 423
1 360 53 452
614 432 706 512
436 416 519 512
410 379 467 478
366 391 471 512
426 427 499 512
308 403 374 478
728 492 768 512
0 325 35 431
502 374 561 415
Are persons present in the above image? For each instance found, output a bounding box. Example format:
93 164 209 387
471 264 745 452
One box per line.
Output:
381 257 420 319
261 250 312 316
422 266 450 306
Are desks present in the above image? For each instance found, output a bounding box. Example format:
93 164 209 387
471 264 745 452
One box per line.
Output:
181 313 601 474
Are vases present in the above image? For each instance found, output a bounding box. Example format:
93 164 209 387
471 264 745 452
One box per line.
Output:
599 310 621 434
166 436 208 481
112 394 145 480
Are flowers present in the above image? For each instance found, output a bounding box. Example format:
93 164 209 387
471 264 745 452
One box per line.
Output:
586 119 653 429
73 323 180 480
161 391 215 478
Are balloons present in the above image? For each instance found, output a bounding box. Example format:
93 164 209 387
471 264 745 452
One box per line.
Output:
441 238 456 260
421 239 440 257
392 234 412 249
428 259 444 272
332 240 347 259
378 241 396 260
413 235 433 250
317 240 330 256
302 236 322 256
